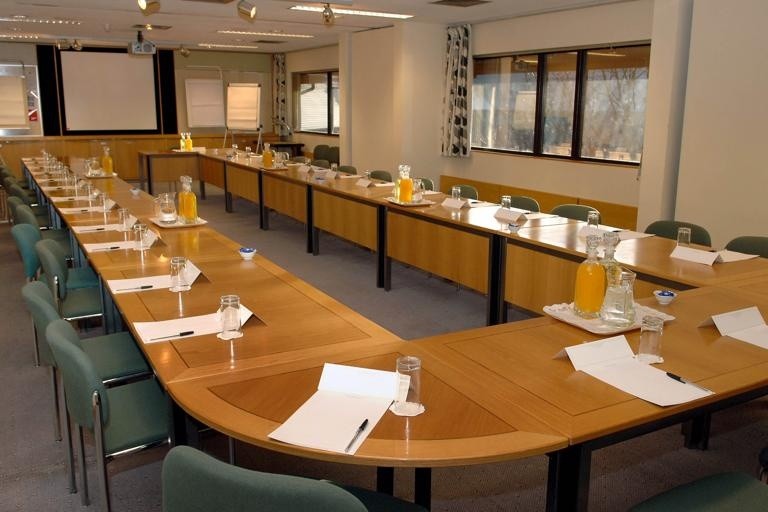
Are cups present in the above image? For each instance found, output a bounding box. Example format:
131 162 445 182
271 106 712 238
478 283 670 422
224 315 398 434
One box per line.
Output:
638 315 664 363
501 195 511 211
170 257 190 292
232 143 371 181
452 186 461 201
43 151 148 251
586 210 599 234
676 226 691 249
395 356 421 415
219 295 243 338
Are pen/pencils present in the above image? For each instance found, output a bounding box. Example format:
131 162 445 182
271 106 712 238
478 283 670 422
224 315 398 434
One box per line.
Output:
613 231 620 232
96 247 120 249
117 286 153 291
666 371 708 391
82 228 104 232
151 331 194 340
710 249 722 252
472 202 479 203
67 209 88 212
524 212 532 214
345 419 368 452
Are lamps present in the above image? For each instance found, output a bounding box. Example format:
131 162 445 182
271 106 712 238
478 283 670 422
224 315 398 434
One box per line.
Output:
236 1 258 19
180 46 192 58
321 4 336 27
72 39 82 53
135 0 161 17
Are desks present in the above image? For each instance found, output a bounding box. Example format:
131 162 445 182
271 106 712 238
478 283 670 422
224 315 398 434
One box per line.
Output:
259 140 304 157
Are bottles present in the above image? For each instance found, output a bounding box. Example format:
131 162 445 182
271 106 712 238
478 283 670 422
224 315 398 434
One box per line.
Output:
394 164 425 204
152 175 198 226
573 232 638 328
180 132 193 153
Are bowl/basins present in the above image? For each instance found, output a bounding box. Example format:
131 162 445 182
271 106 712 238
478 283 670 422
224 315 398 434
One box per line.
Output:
226 156 233 160
238 247 257 260
508 223 521 234
652 289 678 305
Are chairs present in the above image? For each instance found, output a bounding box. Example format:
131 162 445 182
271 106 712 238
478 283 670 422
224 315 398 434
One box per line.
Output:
0 144 764 511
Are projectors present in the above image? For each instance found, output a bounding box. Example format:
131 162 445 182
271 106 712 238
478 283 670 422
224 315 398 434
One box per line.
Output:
127 40 157 55
